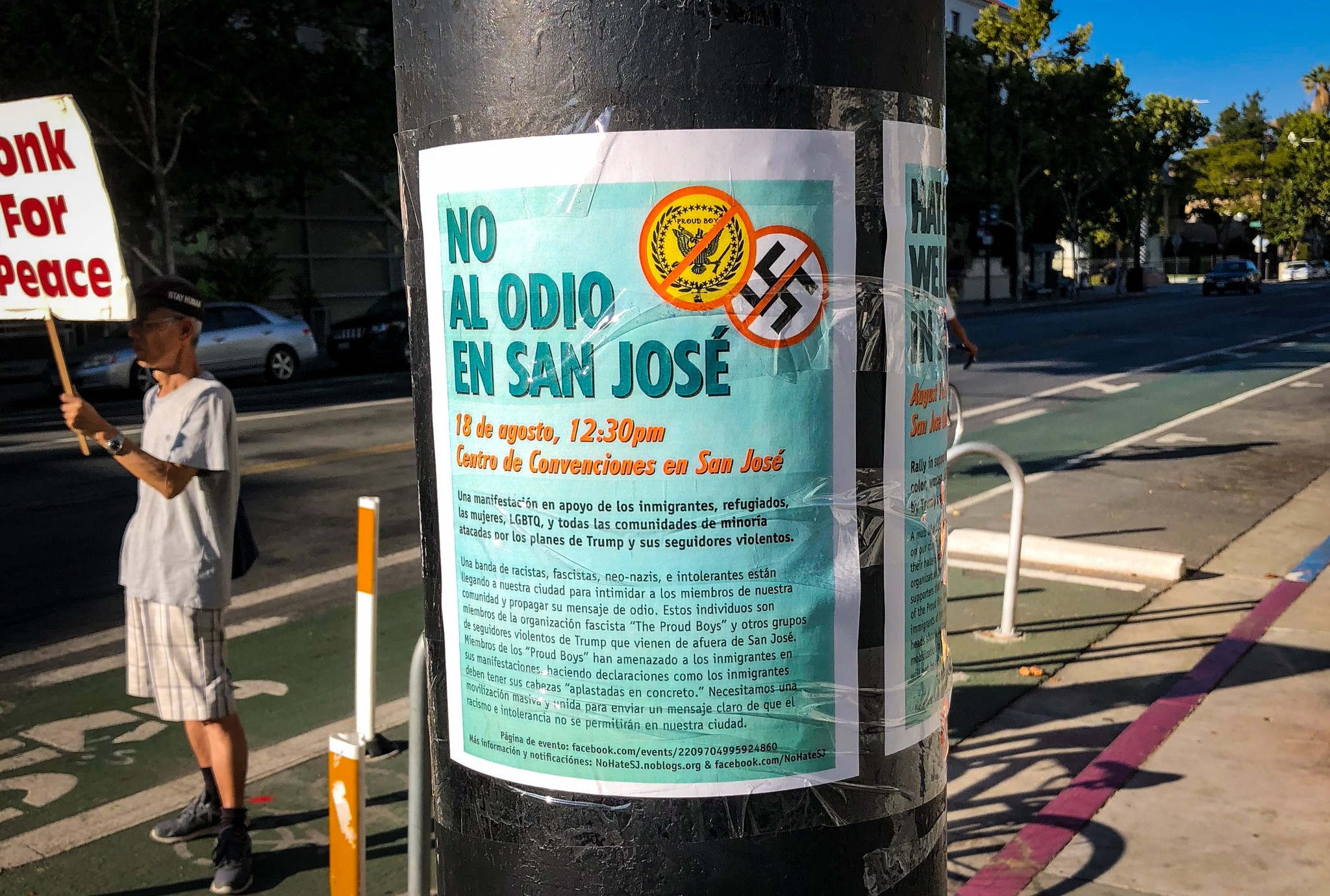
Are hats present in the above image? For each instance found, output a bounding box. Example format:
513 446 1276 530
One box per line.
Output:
133 274 206 319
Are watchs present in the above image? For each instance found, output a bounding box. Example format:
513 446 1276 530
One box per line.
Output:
104 432 127 458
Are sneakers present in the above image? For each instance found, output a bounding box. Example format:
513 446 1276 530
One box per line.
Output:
149 790 223 844
210 823 253 894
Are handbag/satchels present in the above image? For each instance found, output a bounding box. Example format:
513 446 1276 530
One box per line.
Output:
232 494 258 580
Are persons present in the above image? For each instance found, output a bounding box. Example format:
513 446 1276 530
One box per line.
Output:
58 275 259 893
947 293 978 359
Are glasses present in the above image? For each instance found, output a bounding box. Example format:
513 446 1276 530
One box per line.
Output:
128 316 183 329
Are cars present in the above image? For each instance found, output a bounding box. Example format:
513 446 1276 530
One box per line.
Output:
1311 259 1330 280
72 302 321 402
1281 260 1314 282
1201 259 1263 297
324 286 412 370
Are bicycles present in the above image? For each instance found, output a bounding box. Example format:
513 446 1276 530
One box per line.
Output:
946 342 979 453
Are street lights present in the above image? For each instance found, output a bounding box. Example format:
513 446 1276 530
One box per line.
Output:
1258 136 1318 282
